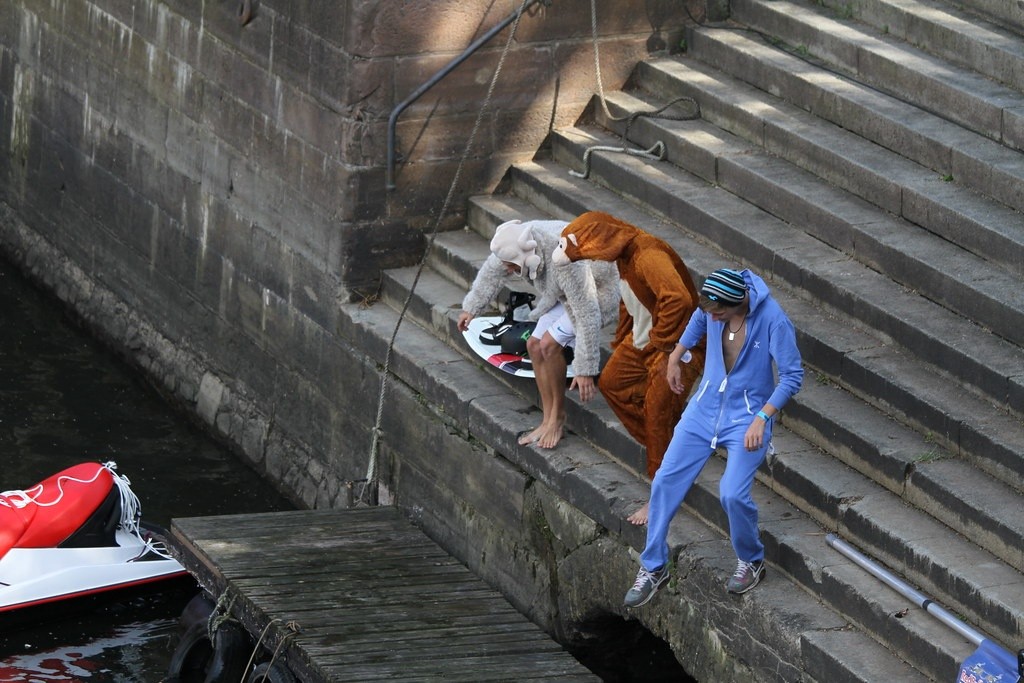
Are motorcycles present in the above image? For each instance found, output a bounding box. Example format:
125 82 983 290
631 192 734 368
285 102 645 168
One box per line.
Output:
0 457 188 626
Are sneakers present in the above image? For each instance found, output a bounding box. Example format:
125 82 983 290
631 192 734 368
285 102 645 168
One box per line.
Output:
520 342 574 369
624 566 672 609
728 557 766 594
479 291 536 345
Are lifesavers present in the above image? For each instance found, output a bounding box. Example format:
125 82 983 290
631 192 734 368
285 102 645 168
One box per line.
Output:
247 662 294 683
168 617 250 683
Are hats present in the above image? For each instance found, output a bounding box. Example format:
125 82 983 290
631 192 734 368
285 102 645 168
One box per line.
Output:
699 267 748 307
490 219 540 280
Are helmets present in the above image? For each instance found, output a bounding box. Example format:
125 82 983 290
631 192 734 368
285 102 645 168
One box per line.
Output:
500 323 536 356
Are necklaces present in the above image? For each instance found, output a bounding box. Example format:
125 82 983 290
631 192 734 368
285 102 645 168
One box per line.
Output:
728 309 749 341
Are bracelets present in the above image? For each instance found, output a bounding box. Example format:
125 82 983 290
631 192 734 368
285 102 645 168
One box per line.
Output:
758 411 769 422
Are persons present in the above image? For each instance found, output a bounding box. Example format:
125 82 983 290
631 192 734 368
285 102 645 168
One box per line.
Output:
624 267 805 608
552 211 707 525
457 218 621 449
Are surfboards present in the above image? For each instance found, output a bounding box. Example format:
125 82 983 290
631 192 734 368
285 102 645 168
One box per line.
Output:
462 315 579 380
